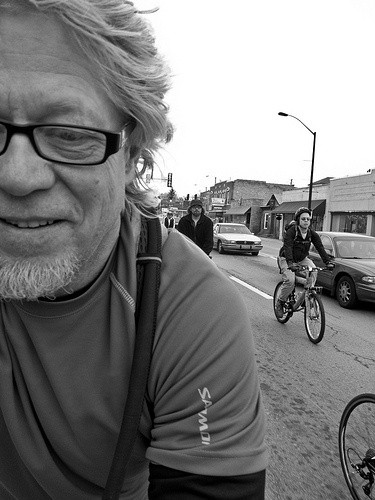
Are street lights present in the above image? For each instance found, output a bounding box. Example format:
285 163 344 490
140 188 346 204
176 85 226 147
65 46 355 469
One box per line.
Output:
206 175 223 223
277 111 316 229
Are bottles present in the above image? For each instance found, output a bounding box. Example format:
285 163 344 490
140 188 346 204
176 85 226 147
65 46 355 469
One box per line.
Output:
295 292 302 302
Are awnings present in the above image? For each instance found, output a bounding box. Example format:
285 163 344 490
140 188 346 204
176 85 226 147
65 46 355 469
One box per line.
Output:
273 200 326 216
224 207 250 215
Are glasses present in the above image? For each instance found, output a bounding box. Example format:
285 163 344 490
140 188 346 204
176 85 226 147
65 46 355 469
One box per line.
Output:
301 217 310 220
192 205 201 209
0 118 137 167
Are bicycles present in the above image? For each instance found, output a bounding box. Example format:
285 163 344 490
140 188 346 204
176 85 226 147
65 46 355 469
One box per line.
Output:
337 392 375 500
273 263 336 344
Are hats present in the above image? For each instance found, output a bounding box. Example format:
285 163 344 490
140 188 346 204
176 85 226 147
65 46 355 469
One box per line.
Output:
167 212 173 217
188 200 205 215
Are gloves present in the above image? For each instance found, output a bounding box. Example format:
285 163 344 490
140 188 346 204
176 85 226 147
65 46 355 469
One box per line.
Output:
288 261 299 272
326 261 334 269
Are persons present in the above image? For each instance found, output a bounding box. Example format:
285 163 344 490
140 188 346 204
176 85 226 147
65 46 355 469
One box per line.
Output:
164 212 175 228
0 0 266 500
276 208 336 317
178 199 214 256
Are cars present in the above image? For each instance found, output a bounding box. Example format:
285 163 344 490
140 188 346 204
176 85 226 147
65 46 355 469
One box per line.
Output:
308 231 375 309
212 223 263 256
174 217 181 226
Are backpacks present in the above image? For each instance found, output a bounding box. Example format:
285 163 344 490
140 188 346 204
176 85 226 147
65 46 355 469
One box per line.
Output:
282 219 312 244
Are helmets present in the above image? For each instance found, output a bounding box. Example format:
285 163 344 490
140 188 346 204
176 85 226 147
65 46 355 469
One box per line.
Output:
294 207 311 221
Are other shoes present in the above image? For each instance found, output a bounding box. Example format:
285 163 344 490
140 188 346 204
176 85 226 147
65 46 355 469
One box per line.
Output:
301 299 314 308
275 300 283 318
